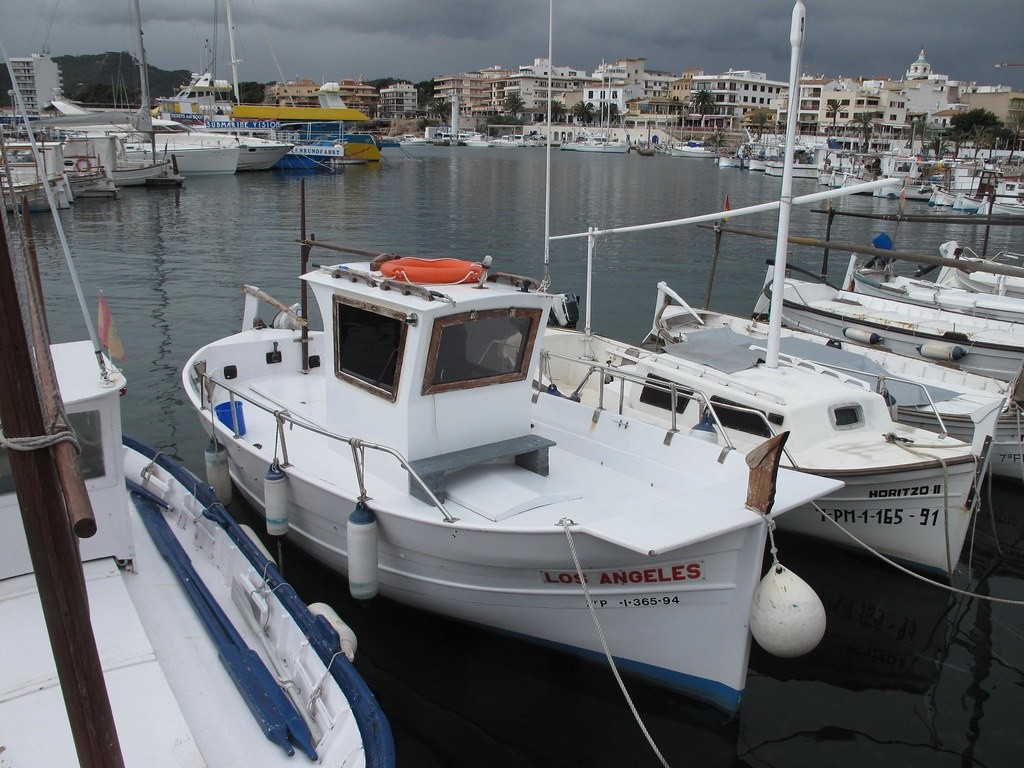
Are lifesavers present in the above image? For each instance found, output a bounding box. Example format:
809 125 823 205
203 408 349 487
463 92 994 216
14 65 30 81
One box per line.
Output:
75 158 91 172
380 256 485 284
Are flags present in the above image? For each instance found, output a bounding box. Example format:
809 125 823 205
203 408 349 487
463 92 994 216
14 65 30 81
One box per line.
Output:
97 290 127 365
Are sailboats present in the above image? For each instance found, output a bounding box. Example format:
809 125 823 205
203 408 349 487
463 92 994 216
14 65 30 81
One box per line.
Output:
496 0 1013 580
0 1 720 215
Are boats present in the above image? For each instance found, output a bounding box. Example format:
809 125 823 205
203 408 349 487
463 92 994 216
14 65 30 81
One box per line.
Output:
1 219 398 768
179 171 851 725
722 124 1024 484
